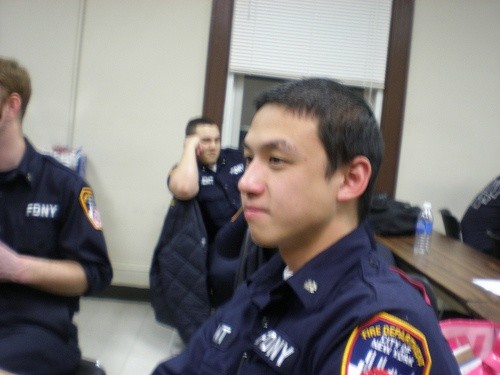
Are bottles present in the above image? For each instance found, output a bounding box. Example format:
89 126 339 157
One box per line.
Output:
413 202 434 256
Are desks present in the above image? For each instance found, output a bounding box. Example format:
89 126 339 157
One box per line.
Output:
374 235 500 304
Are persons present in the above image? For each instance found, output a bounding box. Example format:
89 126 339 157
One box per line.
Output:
151 78 461 375
0 60 113 375
165 118 250 248
